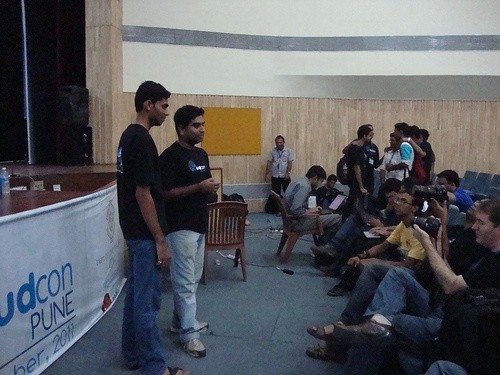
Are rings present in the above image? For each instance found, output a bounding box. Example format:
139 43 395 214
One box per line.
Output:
157 261 163 264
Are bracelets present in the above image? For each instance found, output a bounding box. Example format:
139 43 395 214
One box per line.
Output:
287 172 291 174
358 249 370 260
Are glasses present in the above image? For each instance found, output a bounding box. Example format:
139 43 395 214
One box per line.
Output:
392 198 417 208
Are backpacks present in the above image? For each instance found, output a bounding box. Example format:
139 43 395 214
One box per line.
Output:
399 144 428 185
336 151 355 186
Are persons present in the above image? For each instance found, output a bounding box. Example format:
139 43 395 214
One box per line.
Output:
159 106 219 358
117 80 191 375
306 123 500 375
264 135 294 216
315 174 339 206
281 165 342 258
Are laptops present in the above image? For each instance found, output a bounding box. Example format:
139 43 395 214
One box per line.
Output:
318 192 347 215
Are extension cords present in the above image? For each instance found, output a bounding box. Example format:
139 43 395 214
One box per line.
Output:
270 229 284 233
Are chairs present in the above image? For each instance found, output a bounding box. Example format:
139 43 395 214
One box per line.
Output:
272 190 321 262
202 202 248 284
446 170 500 226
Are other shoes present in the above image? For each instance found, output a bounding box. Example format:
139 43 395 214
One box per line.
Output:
307 321 340 342
169 321 210 335
183 337 206 358
311 242 350 279
304 344 348 363
332 318 396 347
326 284 350 296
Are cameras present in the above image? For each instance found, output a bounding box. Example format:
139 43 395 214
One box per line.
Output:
409 215 440 238
412 183 448 202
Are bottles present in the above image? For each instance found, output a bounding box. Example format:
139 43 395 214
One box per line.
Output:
0 168 10 193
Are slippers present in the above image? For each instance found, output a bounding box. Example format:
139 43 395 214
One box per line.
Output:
165 365 186 375
